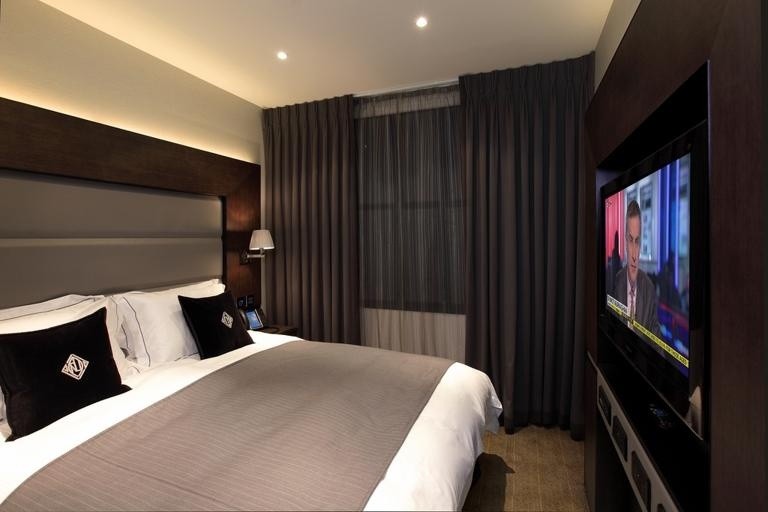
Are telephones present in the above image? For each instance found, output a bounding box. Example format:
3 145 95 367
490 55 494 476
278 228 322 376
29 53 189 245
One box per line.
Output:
238 307 268 330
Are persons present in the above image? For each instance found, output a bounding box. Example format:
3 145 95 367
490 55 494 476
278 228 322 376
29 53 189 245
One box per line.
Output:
613 200 665 357
611 230 620 290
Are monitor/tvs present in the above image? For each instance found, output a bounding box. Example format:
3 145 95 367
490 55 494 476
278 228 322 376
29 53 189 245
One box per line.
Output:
595 131 707 443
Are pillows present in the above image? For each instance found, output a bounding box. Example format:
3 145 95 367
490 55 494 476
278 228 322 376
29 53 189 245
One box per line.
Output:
109 278 226 372
1 294 144 439
177 288 255 361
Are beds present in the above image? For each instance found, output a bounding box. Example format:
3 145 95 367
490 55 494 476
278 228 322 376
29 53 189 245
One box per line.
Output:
0 275 504 512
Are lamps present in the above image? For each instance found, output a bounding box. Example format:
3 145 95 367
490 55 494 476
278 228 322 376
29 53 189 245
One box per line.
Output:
238 229 275 265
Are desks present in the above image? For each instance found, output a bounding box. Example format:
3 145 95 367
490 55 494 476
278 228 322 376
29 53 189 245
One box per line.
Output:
586 348 712 512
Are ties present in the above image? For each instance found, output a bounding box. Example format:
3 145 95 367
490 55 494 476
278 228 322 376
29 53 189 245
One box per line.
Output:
629 281 636 324
